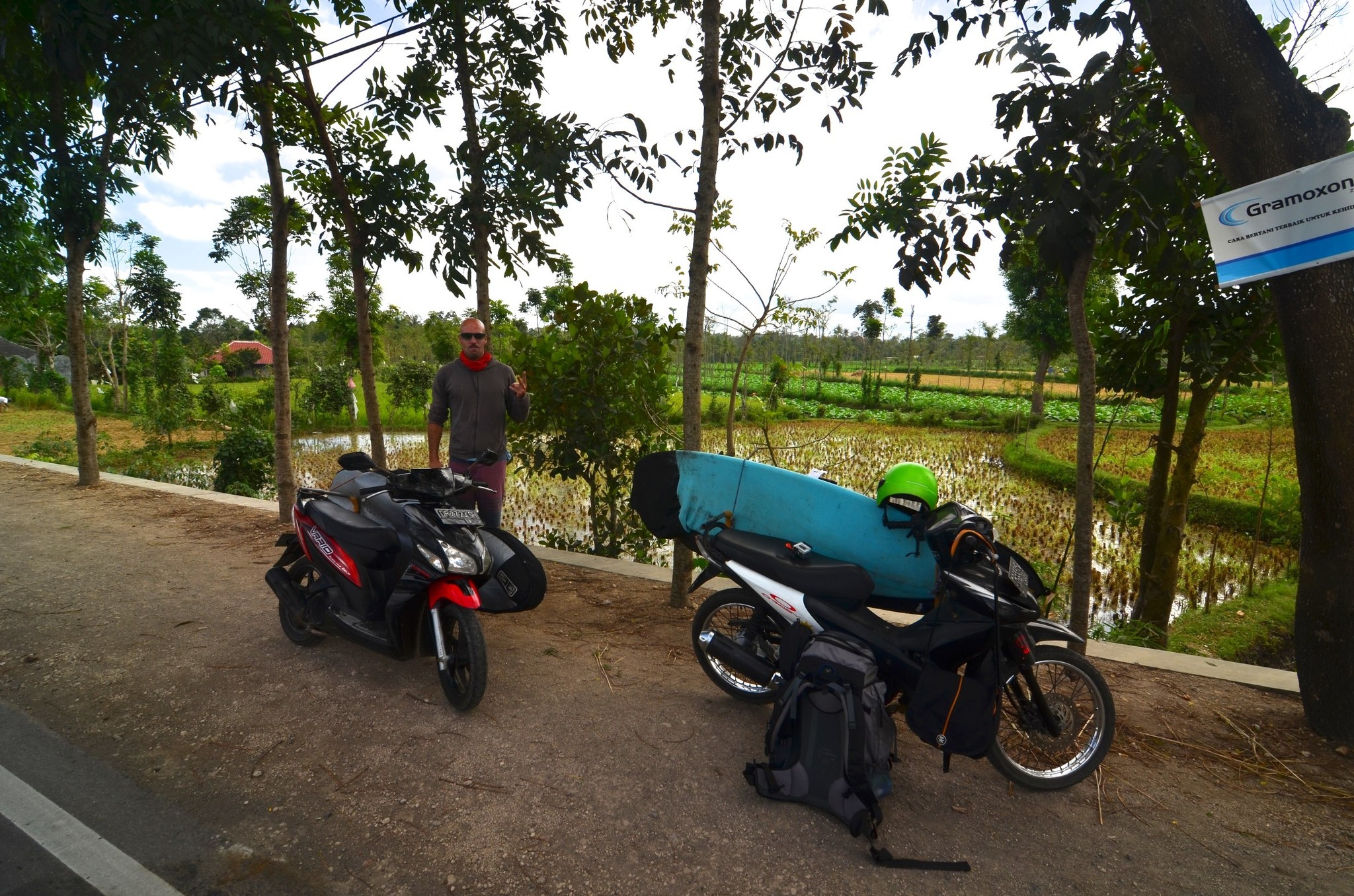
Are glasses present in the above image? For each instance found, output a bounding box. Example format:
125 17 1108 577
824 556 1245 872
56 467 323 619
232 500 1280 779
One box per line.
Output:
460 332 486 341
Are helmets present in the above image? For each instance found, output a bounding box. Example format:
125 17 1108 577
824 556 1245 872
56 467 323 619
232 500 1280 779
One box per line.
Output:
876 462 939 513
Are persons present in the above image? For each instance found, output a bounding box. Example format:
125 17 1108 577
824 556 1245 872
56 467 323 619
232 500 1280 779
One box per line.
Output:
428 318 530 530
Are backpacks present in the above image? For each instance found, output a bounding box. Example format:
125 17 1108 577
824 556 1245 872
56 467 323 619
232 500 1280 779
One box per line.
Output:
741 629 973 874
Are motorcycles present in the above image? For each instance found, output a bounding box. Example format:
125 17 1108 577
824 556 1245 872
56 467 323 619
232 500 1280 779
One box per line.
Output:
686 501 1116 792
264 449 497 712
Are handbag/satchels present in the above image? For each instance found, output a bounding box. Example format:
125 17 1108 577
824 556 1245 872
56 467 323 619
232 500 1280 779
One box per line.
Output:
897 528 1004 774
